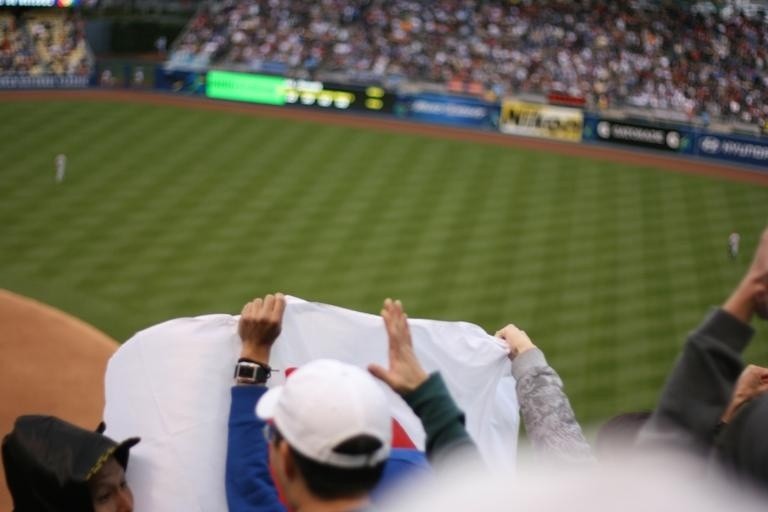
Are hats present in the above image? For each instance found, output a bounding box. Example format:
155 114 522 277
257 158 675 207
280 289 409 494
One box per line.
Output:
256 360 394 467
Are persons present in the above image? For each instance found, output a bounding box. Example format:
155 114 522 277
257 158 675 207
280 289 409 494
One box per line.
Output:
134 64 146 82
0 9 92 78
223 292 481 511
495 321 656 475
727 228 742 260
154 35 169 61
636 227 768 512
99 67 113 87
712 362 768 498
169 1 768 137
53 152 69 184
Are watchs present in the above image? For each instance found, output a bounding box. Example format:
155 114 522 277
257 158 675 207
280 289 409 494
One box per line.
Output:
234 357 280 384
